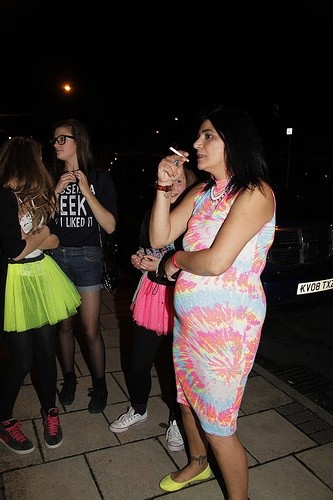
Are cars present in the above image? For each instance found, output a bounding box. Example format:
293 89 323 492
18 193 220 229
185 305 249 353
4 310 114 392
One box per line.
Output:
101 150 333 312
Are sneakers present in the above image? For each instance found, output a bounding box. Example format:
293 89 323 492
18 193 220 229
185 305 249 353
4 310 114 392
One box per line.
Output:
110 406 148 432
59 379 78 405
40 405 64 449
0 417 35 454
165 420 187 451
87 387 108 414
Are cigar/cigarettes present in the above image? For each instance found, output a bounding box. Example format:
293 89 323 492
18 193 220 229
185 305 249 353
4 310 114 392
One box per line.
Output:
170 147 190 162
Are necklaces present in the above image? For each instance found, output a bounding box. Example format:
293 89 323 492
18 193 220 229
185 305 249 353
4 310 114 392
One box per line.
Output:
211 185 225 200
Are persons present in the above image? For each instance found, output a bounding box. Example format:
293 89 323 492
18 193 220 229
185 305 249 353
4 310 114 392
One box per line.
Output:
47 120 118 413
149 107 275 500
0 137 82 454
108 166 202 452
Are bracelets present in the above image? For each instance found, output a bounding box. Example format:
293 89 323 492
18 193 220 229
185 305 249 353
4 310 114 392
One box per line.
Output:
171 250 181 268
154 179 173 192
54 190 61 194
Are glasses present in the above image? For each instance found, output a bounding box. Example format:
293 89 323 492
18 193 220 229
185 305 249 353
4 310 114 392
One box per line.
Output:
49 135 76 146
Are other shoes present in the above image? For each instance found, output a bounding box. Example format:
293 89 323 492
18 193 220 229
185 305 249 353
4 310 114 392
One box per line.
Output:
160 463 211 491
